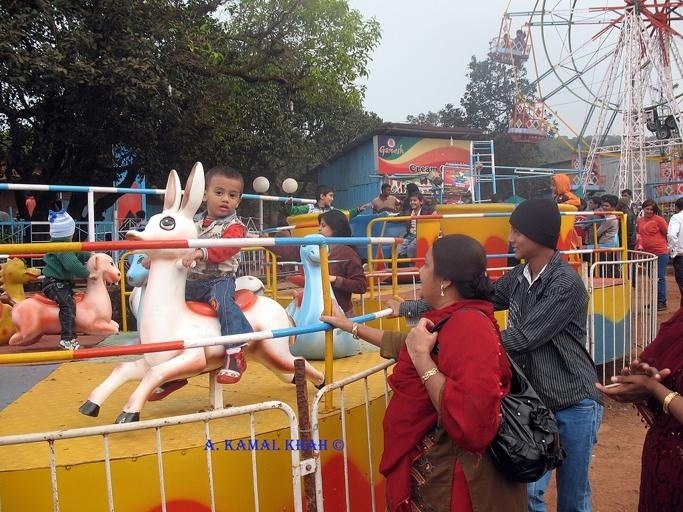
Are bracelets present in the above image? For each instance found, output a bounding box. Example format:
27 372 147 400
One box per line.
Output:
420 367 438 384
663 391 679 414
353 322 359 341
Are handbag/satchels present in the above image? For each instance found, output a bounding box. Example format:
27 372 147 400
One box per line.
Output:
427 307 565 482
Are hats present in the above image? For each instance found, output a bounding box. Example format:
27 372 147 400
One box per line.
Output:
508 197 562 250
48 208 76 240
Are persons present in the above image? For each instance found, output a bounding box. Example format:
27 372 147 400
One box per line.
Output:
143 164 253 402
283 184 368 221
596 195 622 277
388 193 433 270
620 189 637 216
667 198 683 306
504 30 526 50
319 234 531 512
615 197 637 288
402 183 428 209
385 199 603 512
595 306 683 512
552 174 580 207
576 198 587 212
0 257 42 344
247 210 369 319
42 210 99 351
362 182 403 215
573 196 605 275
637 198 669 312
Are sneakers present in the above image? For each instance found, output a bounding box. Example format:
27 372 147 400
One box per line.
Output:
645 302 654 309
217 349 248 384
657 303 667 310
58 339 80 351
147 378 189 403
